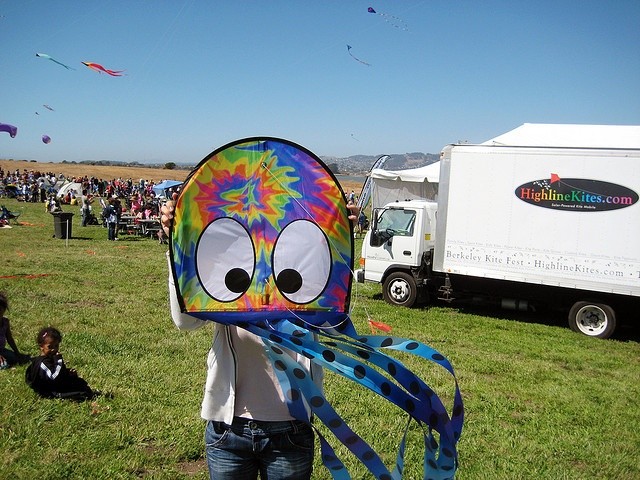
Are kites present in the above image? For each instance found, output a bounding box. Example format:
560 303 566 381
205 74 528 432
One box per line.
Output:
346 44 369 66
0 123 17 138
81 61 128 76
36 53 71 71
43 105 54 111
35 112 39 115
169 136 463 480
41 135 51 144
367 7 407 36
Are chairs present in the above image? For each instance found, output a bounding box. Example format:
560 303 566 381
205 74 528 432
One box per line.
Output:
1 204 20 225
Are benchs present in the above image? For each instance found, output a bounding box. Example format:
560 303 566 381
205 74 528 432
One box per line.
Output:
118 222 134 225
133 226 141 235
126 225 137 230
147 229 161 239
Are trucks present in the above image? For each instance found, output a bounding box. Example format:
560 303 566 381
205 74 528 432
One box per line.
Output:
353 143 640 339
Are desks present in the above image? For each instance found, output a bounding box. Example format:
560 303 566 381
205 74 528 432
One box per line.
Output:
120 216 137 225
137 218 158 235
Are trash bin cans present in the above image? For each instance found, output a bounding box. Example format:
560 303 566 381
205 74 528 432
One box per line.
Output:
48 212 75 239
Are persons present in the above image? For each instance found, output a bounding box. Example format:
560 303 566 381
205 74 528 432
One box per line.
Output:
0 205 11 219
356 196 359 205
82 190 95 227
106 199 119 241
25 327 114 404
345 192 348 199
0 167 182 206
158 197 169 245
119 206 159 240
114 200 122 240
0 294 32 369
350 190 355 203
0 213 10 225
160 192 360 480
358 207 366 236
